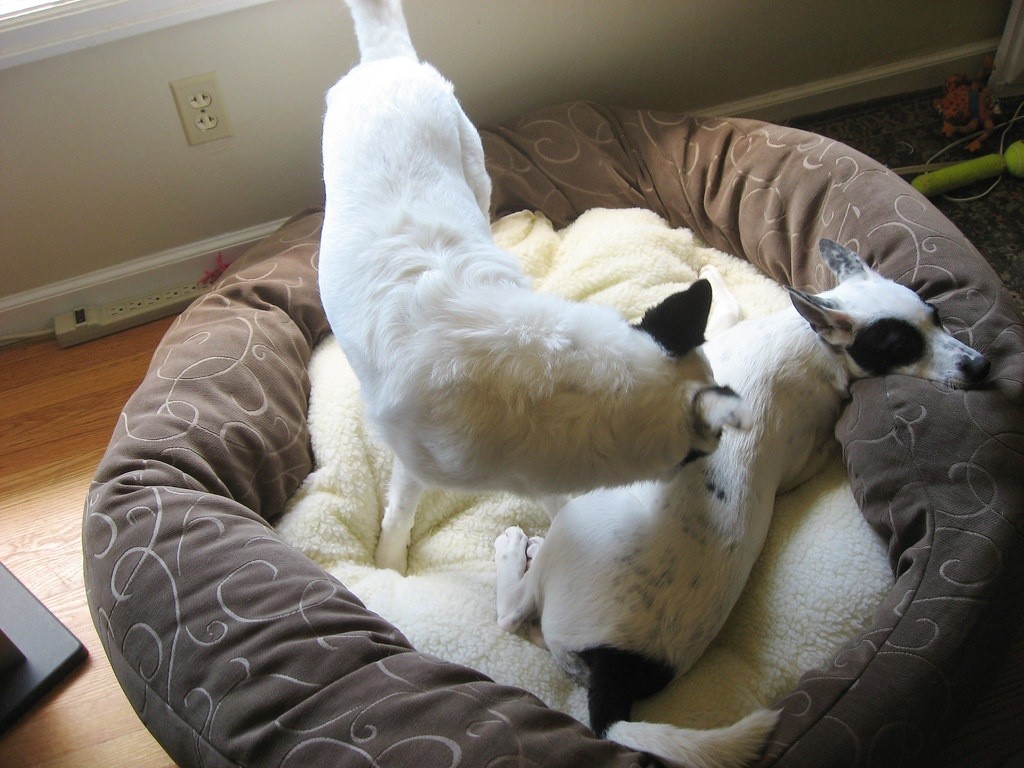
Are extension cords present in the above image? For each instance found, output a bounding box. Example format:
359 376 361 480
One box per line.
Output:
53 279 216 349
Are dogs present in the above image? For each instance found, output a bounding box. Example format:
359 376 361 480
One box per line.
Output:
318 0 989 768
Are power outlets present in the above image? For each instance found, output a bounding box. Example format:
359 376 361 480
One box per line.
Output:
169 70 234 148
